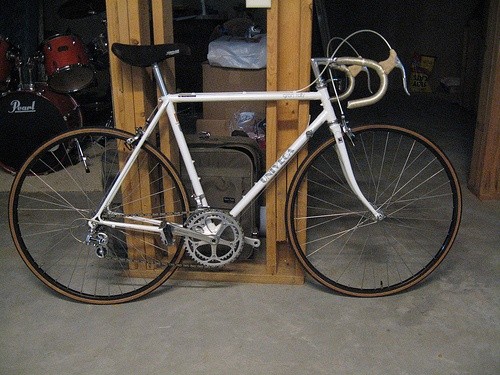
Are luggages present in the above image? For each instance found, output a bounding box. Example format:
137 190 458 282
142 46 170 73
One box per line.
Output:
102 148 128 258
179 134 261 260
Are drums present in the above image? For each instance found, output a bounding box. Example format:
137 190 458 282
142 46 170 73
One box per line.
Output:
39 31 98 95
85 33 111 71
0 36 16 83
0 81 84 177
11 54 49 90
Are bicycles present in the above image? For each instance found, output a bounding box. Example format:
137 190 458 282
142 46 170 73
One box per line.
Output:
8 29 463 305
